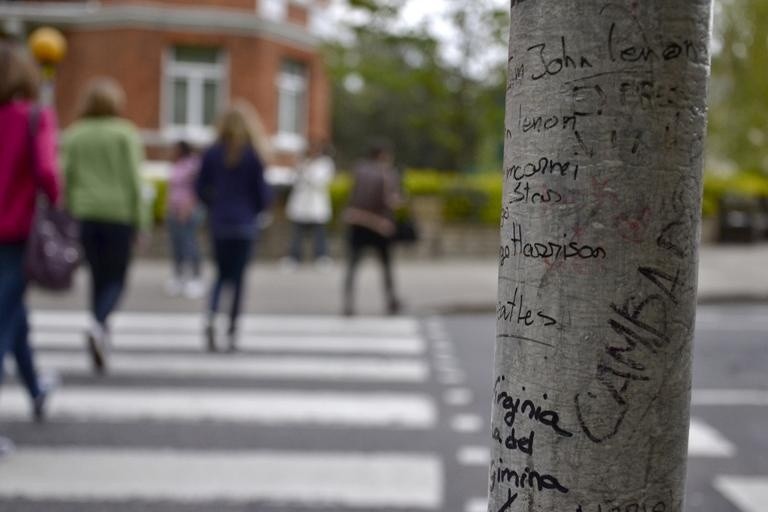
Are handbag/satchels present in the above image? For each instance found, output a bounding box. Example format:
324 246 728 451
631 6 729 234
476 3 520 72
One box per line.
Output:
25 196 75 289
371 214 414 242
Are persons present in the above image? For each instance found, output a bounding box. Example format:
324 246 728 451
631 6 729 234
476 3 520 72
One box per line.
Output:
336 144 405 318
0 45 74 423
283 134 335 274
166 141 206 296
192 100 269 351
55 75 154 379
